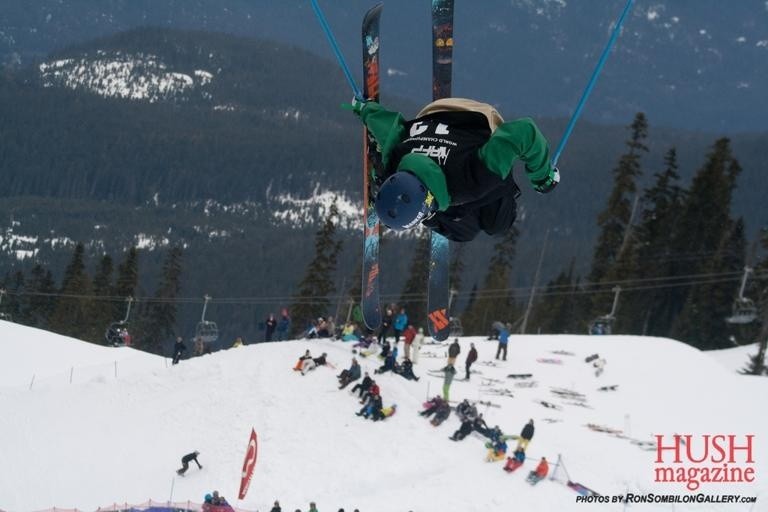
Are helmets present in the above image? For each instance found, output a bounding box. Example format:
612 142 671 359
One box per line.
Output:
374 172 438 231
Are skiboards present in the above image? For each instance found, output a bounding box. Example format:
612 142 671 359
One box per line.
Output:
361 1 456 341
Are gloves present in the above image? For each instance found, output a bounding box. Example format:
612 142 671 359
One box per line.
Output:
351 96 373 119
534 167 560 194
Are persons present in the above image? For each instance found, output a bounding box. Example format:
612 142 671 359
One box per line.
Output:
176 450 203 475
348 94 561 243
172 336 187 364
263 300 549 488
108 326 131 346
201 490 360 512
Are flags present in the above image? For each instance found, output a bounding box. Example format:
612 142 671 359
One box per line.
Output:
237 429 257 499
